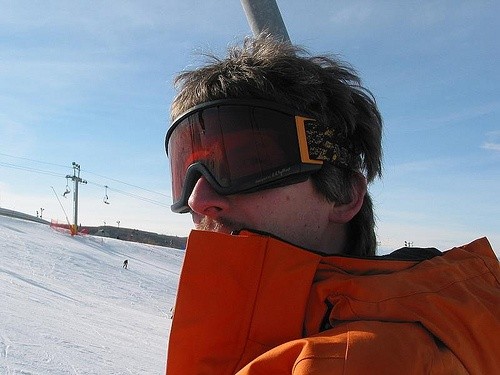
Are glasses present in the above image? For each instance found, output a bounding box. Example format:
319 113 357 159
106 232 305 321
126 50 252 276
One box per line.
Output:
165 97 328 214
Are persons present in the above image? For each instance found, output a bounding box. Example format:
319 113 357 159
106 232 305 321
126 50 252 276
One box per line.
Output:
123 260 127 269
165 33 500 375
